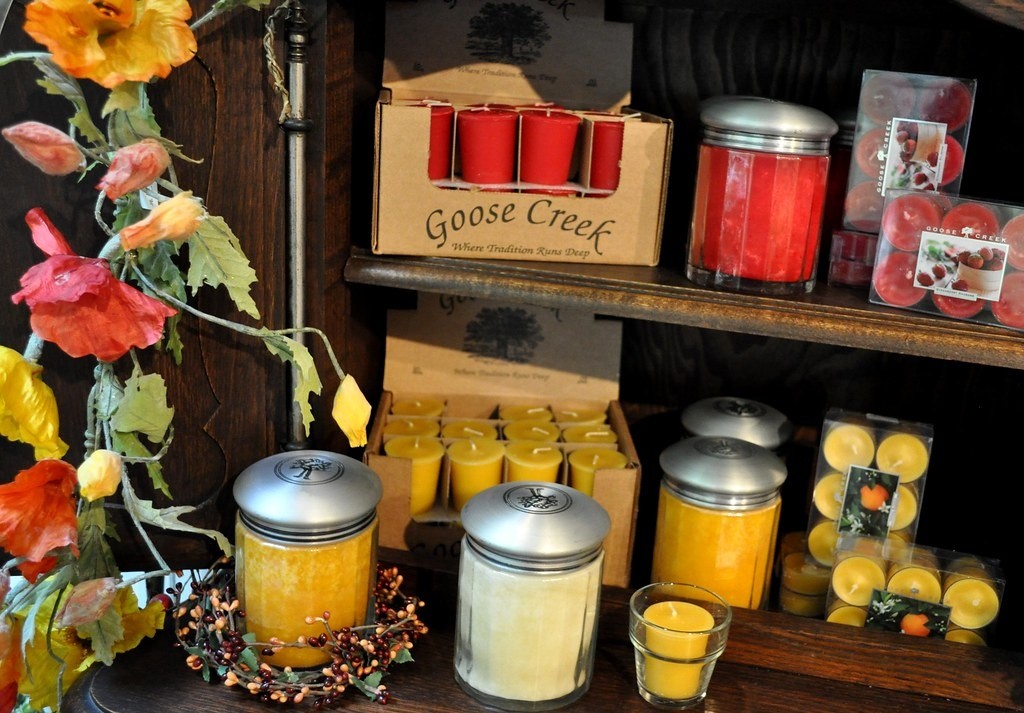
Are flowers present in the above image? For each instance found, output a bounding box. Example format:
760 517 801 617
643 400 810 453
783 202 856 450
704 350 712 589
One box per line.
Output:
0 0 373 713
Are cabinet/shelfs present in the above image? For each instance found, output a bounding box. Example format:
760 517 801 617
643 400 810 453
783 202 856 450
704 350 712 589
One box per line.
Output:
344 239 1024 713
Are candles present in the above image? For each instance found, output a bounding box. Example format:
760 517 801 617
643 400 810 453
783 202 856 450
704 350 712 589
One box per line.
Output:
520 108 582 186
776 411 1000 646
628 580 732 712
405 102 455 180
383 398 628 516
829 73 1024 329
584 112 642 191
457 107 520 185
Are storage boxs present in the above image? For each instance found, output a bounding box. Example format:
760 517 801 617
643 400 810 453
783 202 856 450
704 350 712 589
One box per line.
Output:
361 293 641 587
824 228 878 289
823 530 1007 646
868 188 1024 331
372 0 675 267
802 407 935 572
842 70 977 236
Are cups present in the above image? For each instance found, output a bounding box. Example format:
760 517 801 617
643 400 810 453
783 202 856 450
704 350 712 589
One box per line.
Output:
627 581 732 711
